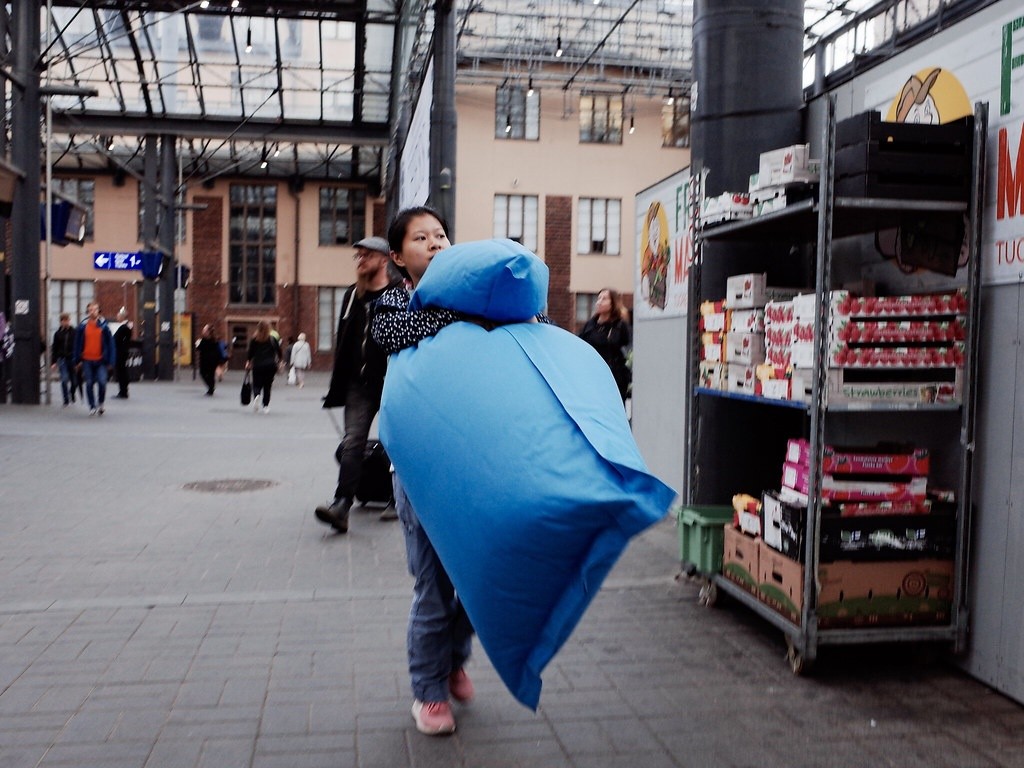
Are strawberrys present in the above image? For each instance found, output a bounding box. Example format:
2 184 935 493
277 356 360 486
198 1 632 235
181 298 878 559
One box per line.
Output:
732 192 750 205
765 305 813 366
743 281 764 378
835 293 966 366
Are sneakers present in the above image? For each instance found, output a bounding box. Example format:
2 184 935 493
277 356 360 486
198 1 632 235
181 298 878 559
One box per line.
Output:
411 697 456 735
449 666 472 701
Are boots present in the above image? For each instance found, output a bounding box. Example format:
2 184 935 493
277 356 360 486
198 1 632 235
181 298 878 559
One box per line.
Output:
315 493 354 533
380 502 397 519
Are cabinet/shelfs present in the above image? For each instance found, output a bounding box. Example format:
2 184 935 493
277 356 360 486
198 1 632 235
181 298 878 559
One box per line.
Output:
672 91 967 674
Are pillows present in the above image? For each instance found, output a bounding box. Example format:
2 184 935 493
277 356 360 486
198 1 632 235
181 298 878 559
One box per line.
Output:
359 234 681 714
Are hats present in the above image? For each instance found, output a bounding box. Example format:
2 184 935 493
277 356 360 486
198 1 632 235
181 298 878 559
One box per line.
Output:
353 236 390 255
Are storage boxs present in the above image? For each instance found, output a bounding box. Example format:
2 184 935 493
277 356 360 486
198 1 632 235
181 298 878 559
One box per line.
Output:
696 142 958 636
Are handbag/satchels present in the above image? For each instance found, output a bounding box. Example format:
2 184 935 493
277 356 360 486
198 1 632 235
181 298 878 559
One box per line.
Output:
287 365 296 384
241 369 251 405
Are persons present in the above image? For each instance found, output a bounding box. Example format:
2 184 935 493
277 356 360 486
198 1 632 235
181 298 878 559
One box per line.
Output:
369 206 558 735
72 303 115 416
51 313 85 406
315 238 392 535
579 288 634 431
283 332 312 389
195 322 228 397
245 318 283 414
113 306 133 399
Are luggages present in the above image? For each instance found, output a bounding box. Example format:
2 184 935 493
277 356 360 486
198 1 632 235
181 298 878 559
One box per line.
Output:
334 439 394 508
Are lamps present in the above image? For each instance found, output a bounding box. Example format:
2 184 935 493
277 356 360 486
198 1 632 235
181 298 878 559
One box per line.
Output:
244 15 254 57
502 22 677 142
260 139 281 168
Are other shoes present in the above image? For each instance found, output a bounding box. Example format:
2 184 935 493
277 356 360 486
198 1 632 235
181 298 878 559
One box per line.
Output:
299 383 305 389
263 406 269 414
89 408 96 416
254 395 261 407
98 407 103 415
